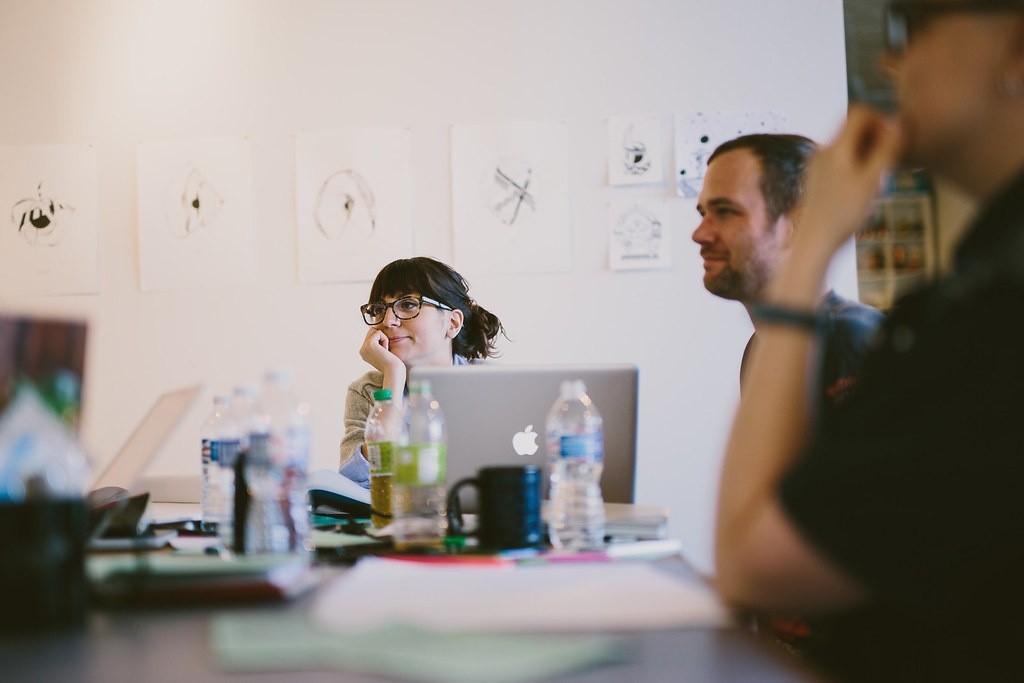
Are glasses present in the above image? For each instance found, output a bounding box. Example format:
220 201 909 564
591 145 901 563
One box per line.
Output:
881 0 1024 58
360 293 465 328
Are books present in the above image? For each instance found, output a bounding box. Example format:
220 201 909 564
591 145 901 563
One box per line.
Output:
88 551 318 602
541 503 670 540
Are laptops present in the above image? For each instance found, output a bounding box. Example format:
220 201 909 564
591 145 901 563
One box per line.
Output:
91 384 204 497
408 366 639 519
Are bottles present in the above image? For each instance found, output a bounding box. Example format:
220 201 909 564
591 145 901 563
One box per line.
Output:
362 390 408 531
545 379 607 550
200 398 242 536
247 371 318 594
403 379 448 537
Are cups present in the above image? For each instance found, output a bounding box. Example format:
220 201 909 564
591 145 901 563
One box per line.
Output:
445 464 542 551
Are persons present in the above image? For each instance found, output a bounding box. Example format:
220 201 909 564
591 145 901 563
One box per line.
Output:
691 135 882 661
716 0 1024 683
339 257 512 490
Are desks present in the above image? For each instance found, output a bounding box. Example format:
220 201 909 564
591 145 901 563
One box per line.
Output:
1 536 838 683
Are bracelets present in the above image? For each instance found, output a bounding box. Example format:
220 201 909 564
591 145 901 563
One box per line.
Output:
751 301 834 336
